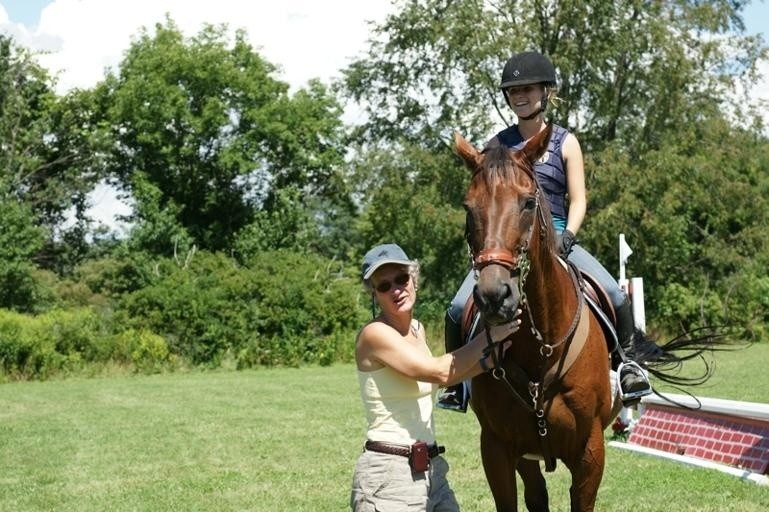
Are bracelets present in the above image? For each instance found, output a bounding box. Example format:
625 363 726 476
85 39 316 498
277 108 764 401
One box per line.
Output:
477 358 493 378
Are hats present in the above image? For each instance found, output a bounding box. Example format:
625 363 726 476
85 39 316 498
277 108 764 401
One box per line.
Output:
362 243 412 279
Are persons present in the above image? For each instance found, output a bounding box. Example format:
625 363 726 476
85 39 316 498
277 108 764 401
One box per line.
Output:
347 243 522 511
435 51 651 409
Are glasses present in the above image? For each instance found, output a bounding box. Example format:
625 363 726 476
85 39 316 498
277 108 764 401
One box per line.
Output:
371 274 409 291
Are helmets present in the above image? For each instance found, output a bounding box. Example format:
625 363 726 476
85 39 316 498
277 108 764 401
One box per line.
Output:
499 52 556 87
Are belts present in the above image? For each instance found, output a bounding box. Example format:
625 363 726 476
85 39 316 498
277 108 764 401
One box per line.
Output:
366 441 445 458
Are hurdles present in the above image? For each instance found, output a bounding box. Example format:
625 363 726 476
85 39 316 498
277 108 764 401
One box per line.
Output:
607 233 768 487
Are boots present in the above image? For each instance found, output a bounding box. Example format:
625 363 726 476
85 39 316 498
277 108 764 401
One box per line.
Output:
438 309 470 406
614 295 650 407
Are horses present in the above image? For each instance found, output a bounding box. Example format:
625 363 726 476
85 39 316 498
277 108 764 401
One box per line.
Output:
448 117 752 511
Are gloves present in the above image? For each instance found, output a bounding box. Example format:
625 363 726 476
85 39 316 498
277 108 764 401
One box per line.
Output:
557 230 575 259
